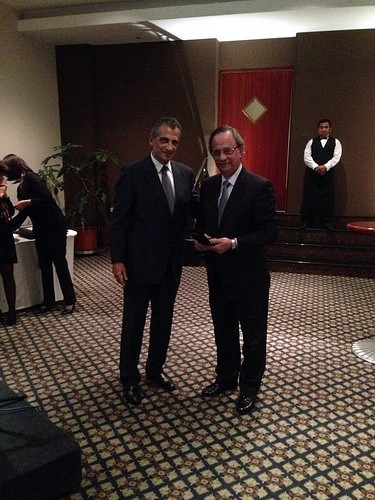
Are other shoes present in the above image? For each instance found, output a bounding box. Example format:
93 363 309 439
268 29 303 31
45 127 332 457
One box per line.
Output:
300 220 310 228
7 308 16 324
324 224 336 232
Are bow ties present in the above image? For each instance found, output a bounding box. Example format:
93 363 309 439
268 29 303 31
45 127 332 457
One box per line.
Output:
320 135 327 141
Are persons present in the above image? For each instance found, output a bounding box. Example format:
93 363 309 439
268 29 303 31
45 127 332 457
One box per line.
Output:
193 124 277 415
297 119 342 232
107 116 201 405
0 153 77 326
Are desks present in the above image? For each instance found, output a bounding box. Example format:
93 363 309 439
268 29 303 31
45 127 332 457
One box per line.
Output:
347 221 375 365
0 362 83 500
0 225 78 314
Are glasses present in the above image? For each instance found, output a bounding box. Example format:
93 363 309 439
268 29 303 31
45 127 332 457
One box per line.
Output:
211 144 239 157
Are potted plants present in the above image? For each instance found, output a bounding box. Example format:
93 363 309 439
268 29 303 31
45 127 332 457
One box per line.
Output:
38 143 123 256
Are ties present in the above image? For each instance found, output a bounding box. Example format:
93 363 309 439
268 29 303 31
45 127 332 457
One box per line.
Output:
218 181 231 227
161 165 174 212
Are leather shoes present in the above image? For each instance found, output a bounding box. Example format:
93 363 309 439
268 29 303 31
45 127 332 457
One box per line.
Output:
124 380 142 404
237 389 257 414
203 382 239 395
145 370 176 390
36 302 55 311
62 302 75 314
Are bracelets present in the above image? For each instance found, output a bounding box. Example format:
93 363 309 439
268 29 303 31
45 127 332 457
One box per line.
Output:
230 239 236 250
